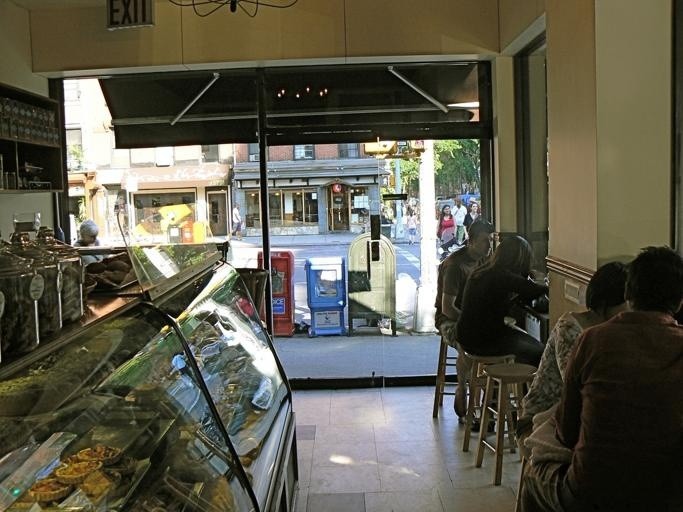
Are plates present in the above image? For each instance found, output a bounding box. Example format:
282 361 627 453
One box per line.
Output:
43 457 153 512
135 417 179 459
61 406 162 468
128 483 203 512
214 384 244 409
216 408 237 433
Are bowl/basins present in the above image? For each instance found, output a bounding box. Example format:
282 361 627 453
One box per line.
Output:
83 279 99 305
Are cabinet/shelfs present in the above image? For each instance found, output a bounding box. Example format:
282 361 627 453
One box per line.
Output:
0 81 65 194
0 258 300 511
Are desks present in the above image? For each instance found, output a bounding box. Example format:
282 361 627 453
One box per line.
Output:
521 302 548 348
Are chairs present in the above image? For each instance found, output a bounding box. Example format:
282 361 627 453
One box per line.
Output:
514 455 530 512
474 363 537 486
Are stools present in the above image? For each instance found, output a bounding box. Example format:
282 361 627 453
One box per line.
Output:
432 331 480 419
462 351 515 453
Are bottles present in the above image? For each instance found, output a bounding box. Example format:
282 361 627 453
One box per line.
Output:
32 225 84 327
0 245 40 358
7 231 64 339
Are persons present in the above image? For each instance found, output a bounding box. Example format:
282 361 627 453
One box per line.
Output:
516 261 634 465
228 202 242 240
451 199 468 242
436 204 455 240
463 202 480 240
434 217 498 432
73 221 99 246
520 244 683 512
456 235 549 396
407 210 417 244
